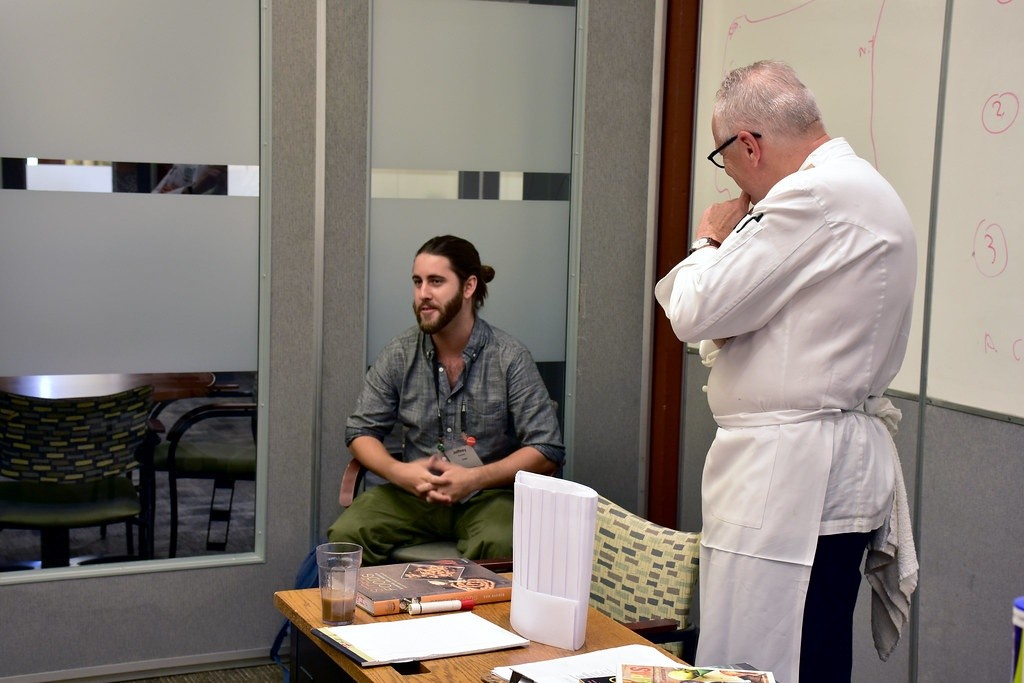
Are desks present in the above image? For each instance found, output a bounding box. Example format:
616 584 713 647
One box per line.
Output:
0 373 216 570
275 572 694 683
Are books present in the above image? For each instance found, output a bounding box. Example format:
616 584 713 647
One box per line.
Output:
310 610 530 666
327 558 513 617
579 662 781 683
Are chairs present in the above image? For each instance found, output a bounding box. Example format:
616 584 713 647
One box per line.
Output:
150 387 259 555
339 455 461 562
0 389 150 572
474 498 705 668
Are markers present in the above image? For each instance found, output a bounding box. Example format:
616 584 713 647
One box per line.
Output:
408 599 475 615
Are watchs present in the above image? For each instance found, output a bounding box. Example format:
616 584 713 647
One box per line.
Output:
688 236 722 255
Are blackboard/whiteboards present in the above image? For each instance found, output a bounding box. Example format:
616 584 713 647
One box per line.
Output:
680 4 1023 426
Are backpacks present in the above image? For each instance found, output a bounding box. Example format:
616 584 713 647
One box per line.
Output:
269 541 328 683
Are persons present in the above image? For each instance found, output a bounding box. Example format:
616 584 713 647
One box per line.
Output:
325 235 567 574
150 164 228 198
654 62 918 683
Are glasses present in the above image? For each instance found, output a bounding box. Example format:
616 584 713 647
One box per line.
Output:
707 132 762 168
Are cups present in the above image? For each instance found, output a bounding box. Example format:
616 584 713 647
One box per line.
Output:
316 541 363 626
